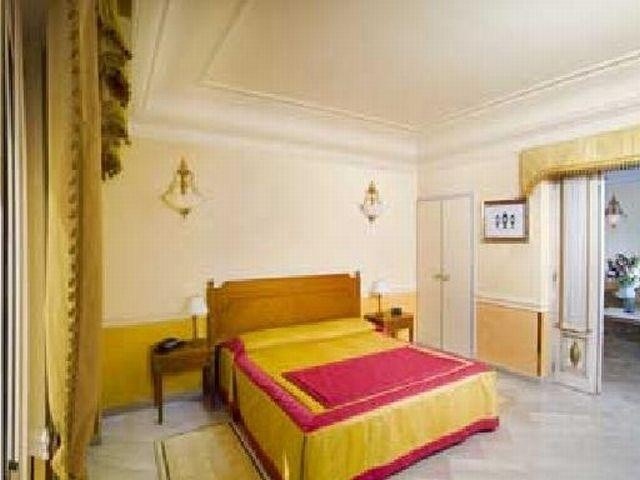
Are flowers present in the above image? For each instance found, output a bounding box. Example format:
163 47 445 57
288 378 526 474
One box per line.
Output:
602 245 639 293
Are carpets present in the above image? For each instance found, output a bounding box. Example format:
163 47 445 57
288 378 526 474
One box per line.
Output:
151 414 271 480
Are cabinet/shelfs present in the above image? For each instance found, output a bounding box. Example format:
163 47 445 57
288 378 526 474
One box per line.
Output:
603 280 640 308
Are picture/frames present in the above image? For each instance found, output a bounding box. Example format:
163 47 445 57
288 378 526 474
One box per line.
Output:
479 193 530 245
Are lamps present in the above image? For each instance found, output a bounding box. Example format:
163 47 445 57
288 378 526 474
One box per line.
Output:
370 277 392 318
158 153 211 223
356 177 389 227
602 188 631 231
182 288 211 351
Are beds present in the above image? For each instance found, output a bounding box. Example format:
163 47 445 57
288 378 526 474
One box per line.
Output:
201 266 507 479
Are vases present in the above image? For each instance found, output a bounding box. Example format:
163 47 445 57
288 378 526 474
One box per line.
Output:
614 280 637 313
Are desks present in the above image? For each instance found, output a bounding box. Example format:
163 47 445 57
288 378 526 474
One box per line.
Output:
361 307 415 347
148 335 223 428
602 301 640 356
